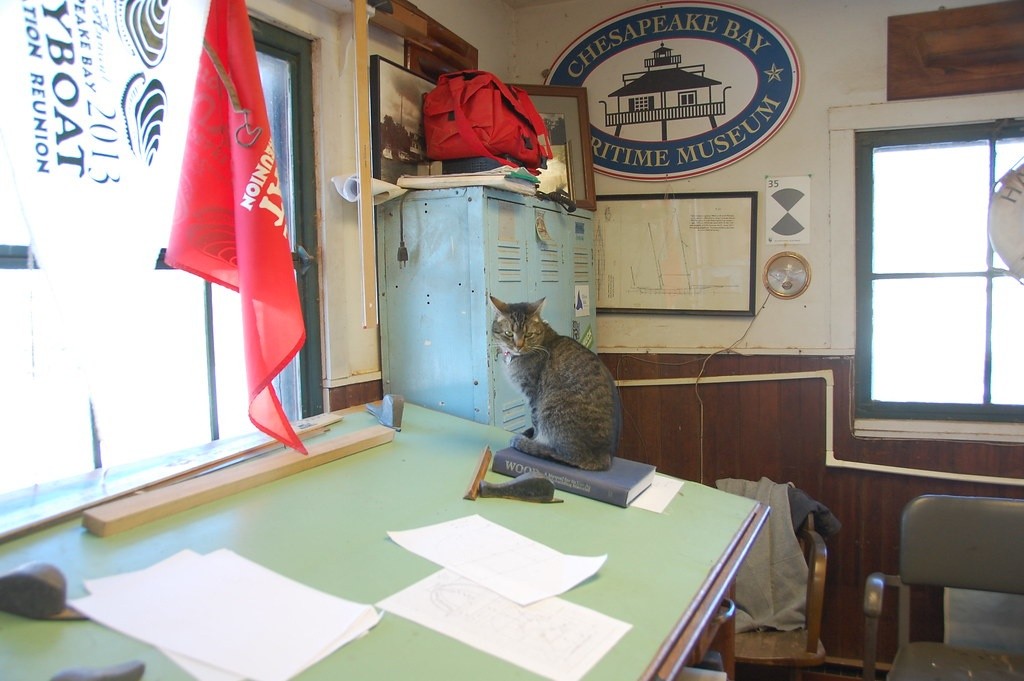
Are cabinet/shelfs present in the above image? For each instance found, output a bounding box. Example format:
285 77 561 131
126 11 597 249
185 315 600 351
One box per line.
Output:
373 187 600 434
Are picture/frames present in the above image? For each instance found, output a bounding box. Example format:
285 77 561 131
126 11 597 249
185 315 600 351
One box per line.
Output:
371 54 437 185
502 85 598 210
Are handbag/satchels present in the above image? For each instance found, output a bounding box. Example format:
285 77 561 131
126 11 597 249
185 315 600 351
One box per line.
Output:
421 70 553 176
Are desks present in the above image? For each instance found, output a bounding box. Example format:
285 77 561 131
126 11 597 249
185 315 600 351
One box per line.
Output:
0 391 774 681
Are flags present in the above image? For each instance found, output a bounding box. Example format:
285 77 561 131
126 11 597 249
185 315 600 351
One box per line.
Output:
166 0 308 456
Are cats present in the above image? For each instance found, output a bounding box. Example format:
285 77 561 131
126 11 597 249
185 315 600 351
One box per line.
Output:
490 295 622 472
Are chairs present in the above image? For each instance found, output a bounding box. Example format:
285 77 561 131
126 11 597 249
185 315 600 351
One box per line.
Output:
731 484 827 681
865 492 1024 681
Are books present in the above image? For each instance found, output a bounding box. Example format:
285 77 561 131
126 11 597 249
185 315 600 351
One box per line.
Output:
492 445 657 508
